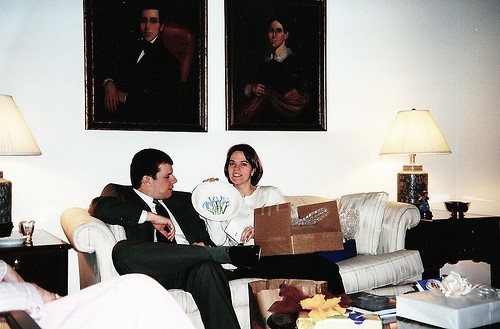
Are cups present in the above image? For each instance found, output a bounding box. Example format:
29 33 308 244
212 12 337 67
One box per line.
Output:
20 221 35 243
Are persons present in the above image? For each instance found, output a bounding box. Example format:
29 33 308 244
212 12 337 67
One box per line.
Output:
202 143 344 296
87 148 261 329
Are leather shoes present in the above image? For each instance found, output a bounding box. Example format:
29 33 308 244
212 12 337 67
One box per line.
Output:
230 245 262 268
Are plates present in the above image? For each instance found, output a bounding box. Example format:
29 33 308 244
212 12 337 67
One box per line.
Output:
0 236 27 244
191 181 242 221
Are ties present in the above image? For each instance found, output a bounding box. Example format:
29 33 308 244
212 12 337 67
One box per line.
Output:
153 200 177 244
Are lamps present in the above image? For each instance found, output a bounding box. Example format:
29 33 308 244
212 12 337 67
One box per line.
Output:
379 108 452 214
0 94 42 237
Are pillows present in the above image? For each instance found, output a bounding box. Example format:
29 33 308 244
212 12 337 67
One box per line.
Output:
338 192 389 254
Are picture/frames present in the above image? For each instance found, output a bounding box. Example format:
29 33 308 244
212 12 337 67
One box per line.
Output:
83 0 208 132
224 0 327 131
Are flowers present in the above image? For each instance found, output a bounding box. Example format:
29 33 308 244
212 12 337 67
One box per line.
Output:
441 271 472 296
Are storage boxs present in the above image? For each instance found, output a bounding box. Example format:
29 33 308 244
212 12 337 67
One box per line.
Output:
253 201 344 256
396 285 500 329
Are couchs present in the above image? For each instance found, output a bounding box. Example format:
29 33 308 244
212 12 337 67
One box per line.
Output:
60 184 423 329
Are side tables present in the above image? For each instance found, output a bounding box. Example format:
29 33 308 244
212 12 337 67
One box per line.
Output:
404 210 500 290
0 229 72 297
